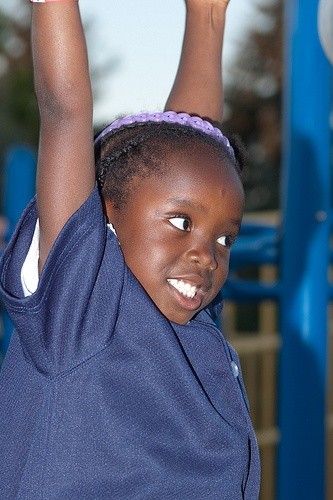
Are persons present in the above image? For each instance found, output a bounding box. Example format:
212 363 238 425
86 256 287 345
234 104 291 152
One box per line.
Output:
0 0 262 499
